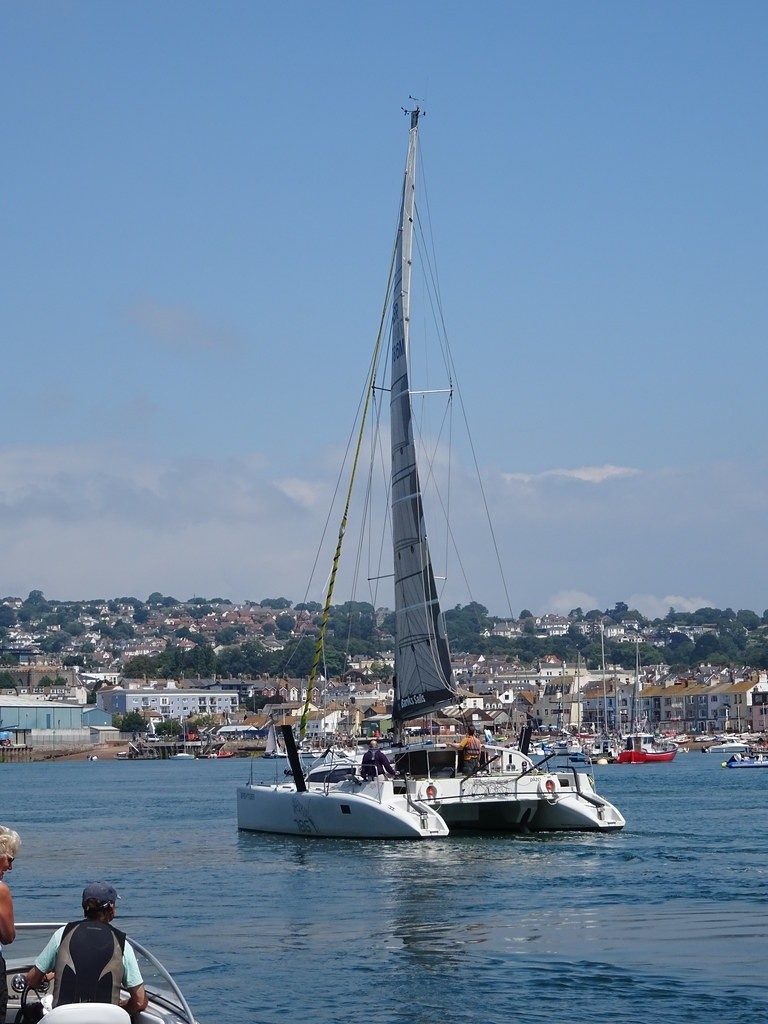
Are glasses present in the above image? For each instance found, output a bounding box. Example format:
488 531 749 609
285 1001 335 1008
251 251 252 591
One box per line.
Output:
6 852 15 863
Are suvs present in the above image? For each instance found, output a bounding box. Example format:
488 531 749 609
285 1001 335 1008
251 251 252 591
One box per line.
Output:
409 729 429 736
178 733 188 741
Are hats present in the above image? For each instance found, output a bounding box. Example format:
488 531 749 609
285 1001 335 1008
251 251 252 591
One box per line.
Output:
82 881 121 911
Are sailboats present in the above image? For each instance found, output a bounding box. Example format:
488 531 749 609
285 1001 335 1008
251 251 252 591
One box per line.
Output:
168 718 196 760
238 96 627 838
262 715 370 759
505 620 768 763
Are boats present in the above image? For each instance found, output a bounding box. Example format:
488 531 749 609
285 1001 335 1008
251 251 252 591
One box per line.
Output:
721 753 768 770
703 737 749 752
659 732 768 744
0 921 200 1023
197 751 234 757
114 747 159 760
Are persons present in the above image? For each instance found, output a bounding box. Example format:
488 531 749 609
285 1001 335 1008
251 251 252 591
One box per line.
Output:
446 727 481 775
0 825 22 1024
361 740 398 781
26 882 148 1015
